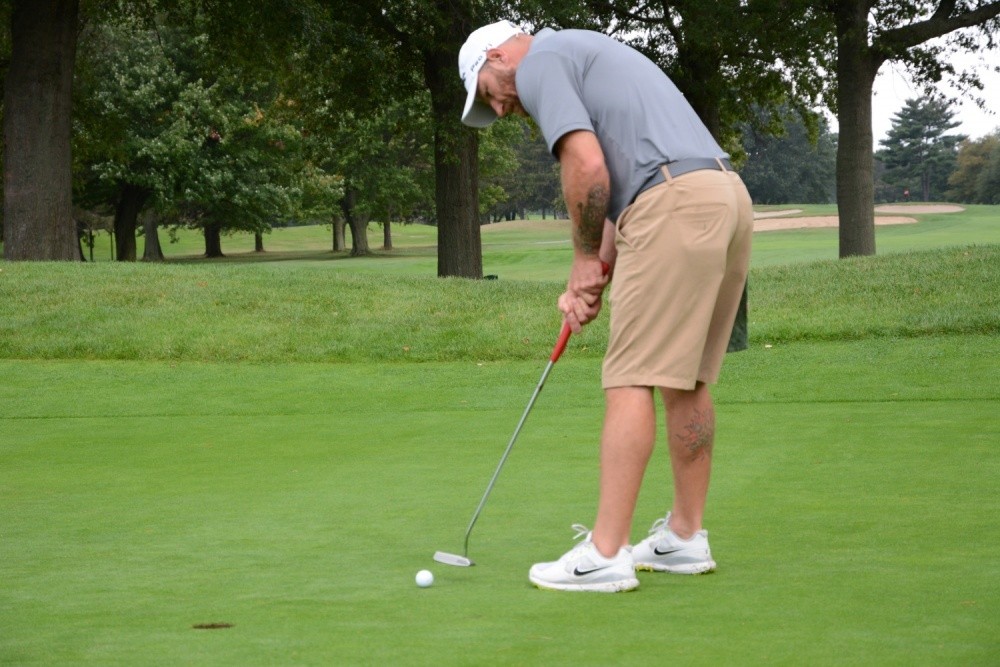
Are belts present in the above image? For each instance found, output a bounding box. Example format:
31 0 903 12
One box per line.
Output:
636 157 736 190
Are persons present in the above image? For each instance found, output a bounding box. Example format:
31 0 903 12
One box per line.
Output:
458 19 754 596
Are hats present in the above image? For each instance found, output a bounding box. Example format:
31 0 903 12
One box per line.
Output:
458 18 526 128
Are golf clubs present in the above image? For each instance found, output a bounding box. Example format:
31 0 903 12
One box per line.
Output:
430 260 615 567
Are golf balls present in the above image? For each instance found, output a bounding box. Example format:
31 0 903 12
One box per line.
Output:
415 569 433 587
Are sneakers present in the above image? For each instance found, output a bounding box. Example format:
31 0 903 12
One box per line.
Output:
634 514 714 574
529 524 640 593
